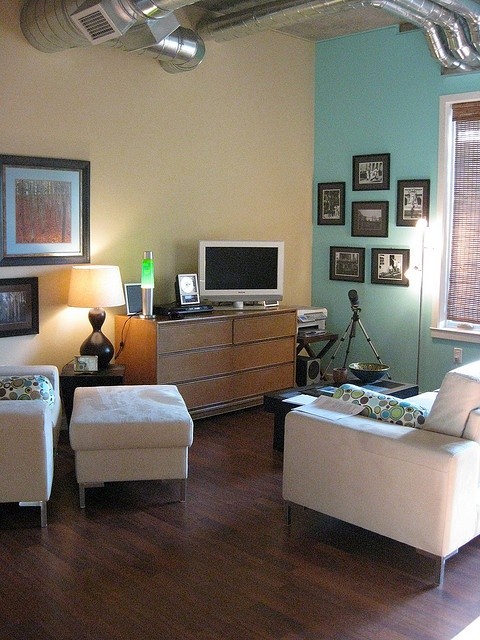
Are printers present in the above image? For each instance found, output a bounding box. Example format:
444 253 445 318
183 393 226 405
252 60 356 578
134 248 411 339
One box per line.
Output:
296 306 328 340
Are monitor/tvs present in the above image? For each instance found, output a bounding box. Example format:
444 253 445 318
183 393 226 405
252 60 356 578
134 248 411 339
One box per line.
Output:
197 240 284 312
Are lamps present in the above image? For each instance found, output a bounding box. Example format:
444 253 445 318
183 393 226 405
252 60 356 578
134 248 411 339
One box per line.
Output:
64 262 129 372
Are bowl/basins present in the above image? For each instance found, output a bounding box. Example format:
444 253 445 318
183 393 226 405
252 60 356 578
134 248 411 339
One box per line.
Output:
349 362 390 383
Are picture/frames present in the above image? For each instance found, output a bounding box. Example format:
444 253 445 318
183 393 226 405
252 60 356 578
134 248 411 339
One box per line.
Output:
369 244 413 288
123 279 155 317
396 177 431 230
349 198 391 239
351 151 392 192
327 245 366 284
177 273 201 305
316 180 347 229
0 151 92 269
0 275 39 339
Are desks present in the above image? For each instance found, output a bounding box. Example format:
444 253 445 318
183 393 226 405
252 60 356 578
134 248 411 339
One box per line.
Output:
60 357 126 431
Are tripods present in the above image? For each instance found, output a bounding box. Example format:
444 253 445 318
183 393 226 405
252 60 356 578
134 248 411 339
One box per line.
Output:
321 307 393 382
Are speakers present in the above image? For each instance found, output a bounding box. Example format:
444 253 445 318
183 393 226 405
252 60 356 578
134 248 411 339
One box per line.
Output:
173 272 200 308
296 356 322 386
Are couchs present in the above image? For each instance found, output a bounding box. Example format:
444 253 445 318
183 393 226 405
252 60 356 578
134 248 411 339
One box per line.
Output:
66 383 196 509
0 362 66 531
280 353 480 590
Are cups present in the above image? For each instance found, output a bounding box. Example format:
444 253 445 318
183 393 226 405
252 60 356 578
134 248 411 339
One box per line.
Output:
333 368 348 384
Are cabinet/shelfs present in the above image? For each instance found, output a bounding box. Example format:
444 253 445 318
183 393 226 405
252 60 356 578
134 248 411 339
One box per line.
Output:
298 331 340 385
112 299 300 426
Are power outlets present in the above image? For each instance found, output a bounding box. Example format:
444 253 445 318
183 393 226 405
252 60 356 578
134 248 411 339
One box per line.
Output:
452 349 463 364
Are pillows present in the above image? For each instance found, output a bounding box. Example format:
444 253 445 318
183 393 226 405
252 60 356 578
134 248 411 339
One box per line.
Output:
332 382 432 430
0 375 56 411
